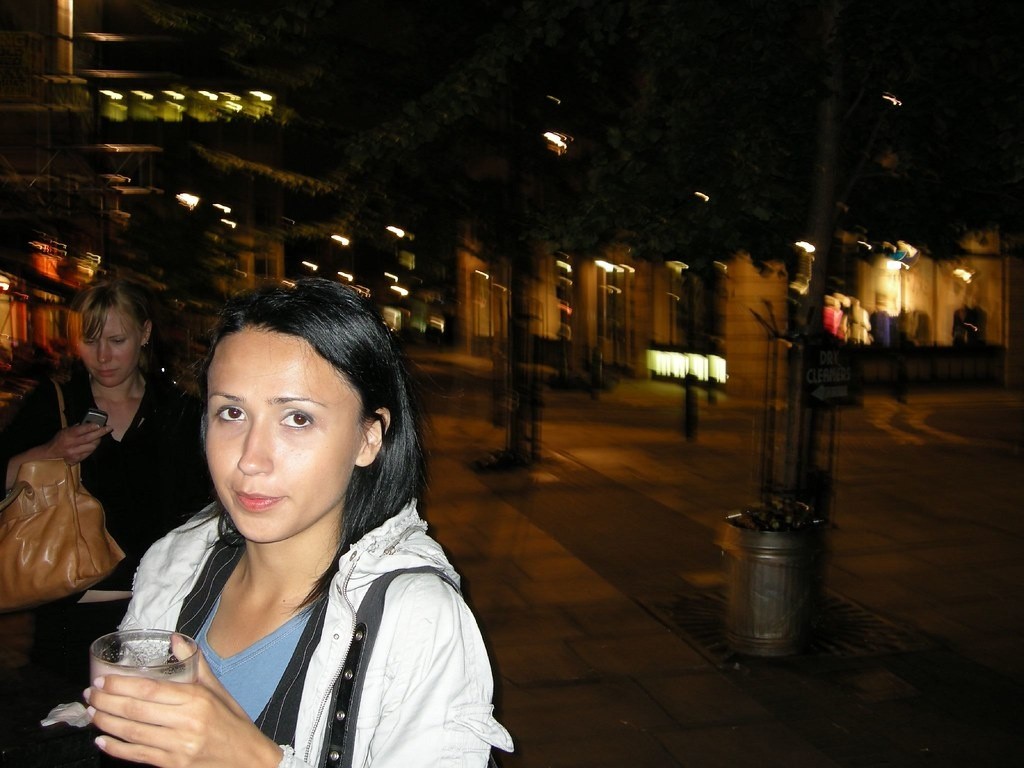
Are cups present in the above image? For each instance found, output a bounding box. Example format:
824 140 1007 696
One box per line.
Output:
89 628 199 688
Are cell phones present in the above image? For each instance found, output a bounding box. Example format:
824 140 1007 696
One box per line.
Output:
79 408 108 428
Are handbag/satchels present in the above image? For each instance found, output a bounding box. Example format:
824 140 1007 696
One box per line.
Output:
0 377 127 612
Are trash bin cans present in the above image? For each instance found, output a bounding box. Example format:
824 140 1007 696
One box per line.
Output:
716 512 826 659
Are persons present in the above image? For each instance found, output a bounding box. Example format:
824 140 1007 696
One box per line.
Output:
86 276 514 768
0 278 215 711
